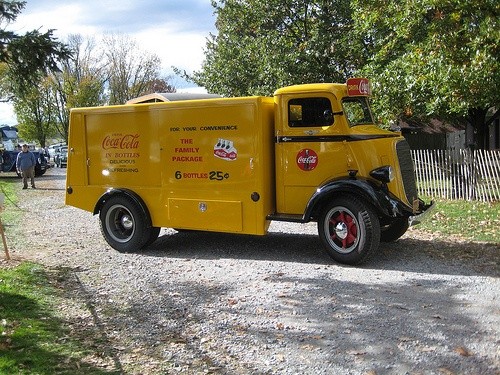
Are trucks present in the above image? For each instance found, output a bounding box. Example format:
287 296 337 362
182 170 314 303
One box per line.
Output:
62 77 435 263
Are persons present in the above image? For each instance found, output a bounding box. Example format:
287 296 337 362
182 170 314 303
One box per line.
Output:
16 144 36 189
15 144 22 151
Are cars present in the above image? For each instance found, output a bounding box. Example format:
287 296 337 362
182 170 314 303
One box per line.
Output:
54 146 68 168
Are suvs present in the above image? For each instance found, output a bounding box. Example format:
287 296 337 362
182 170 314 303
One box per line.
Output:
1 125 52 177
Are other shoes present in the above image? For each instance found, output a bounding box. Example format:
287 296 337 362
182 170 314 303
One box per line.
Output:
22 186 28 189
32 185 36 189
